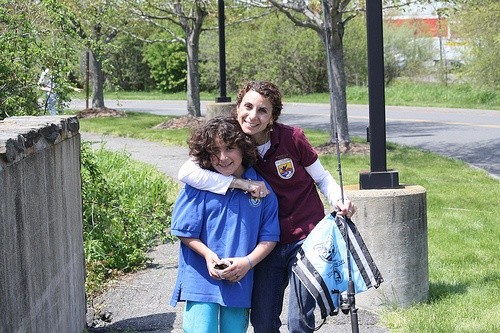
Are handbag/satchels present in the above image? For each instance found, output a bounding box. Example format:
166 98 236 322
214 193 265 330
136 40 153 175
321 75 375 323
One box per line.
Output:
291 210 385 331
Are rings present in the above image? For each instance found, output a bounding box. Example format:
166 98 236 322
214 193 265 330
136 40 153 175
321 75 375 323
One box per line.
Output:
235 274 238 278
349 210 353 213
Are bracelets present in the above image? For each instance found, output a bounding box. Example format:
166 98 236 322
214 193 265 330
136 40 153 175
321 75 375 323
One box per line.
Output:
242 178 252 194
230 177 236 192
245 256 253 268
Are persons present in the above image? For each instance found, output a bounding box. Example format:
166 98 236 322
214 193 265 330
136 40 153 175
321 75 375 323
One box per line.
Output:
38 66 58 116
178 81 357 333
171 113 280 333
